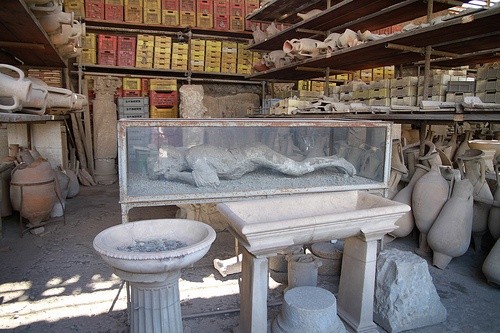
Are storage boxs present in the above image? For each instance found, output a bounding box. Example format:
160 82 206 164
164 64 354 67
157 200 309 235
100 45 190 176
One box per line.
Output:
64 0 500 157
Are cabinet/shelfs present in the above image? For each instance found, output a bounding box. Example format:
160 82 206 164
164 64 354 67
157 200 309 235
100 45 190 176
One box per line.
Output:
0 0 70 147
244 0 500 211
69 17 370 86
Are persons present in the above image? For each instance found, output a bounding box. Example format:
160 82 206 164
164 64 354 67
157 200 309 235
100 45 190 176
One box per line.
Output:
147 144 357 187
